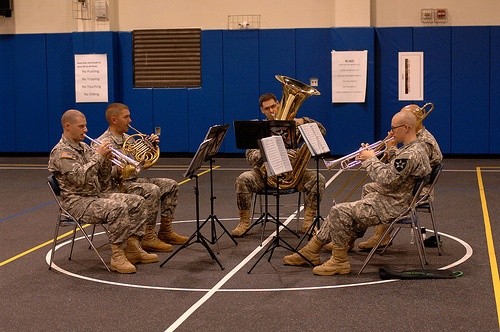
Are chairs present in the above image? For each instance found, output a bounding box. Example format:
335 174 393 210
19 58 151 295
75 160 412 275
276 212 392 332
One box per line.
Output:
358 162 444 276
252 188 302 233
47 174 112 273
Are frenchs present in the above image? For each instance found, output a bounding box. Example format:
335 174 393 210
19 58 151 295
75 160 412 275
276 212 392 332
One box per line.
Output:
122 124 161 170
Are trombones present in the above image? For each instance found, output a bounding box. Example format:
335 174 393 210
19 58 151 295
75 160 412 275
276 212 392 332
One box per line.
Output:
332 102 435 204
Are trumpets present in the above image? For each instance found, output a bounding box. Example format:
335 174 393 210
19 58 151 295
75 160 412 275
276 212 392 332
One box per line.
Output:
322 137 396 172
82 134 142 174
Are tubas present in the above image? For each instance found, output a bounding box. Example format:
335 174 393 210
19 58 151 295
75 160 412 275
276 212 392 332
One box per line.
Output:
252 74 327 190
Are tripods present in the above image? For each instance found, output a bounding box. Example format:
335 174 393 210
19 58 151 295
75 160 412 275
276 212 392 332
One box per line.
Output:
158 120 332 274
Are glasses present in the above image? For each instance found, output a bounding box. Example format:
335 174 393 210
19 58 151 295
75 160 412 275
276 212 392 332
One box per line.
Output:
390 124 410 132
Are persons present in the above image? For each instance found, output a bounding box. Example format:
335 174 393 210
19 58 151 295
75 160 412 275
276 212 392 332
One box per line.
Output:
232 94 327 235
285 104 441 275
93 103 191 251
50 108 160 274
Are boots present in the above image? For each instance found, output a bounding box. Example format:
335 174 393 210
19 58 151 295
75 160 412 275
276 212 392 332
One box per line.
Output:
313 247 351 275
301 208 318 235
358 222 393 250
232 210 252 236
140 224 173 252
282 235 322 266
108 243 136 274
125 236 159 264
324 239 355 251
157 221 189 245
